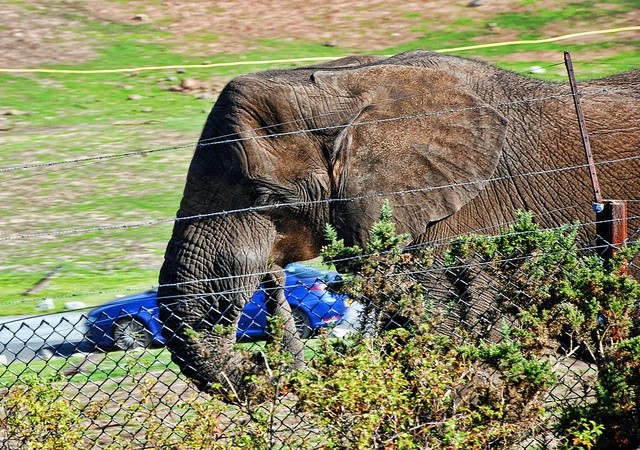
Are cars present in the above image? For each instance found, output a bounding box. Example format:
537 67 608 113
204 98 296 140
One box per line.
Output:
82 263 352 352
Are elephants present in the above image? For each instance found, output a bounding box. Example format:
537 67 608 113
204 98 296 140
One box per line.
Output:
155 47 640 406
